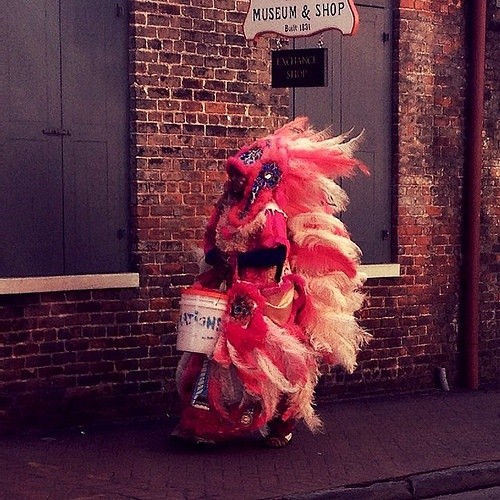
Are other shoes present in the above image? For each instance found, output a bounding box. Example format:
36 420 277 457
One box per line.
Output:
267 432 295 449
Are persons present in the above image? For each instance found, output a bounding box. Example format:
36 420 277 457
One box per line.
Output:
171 137 306 448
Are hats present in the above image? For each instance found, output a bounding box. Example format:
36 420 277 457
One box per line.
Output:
227 136 288 191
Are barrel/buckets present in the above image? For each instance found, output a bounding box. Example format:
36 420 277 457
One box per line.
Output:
175 288 230 355
